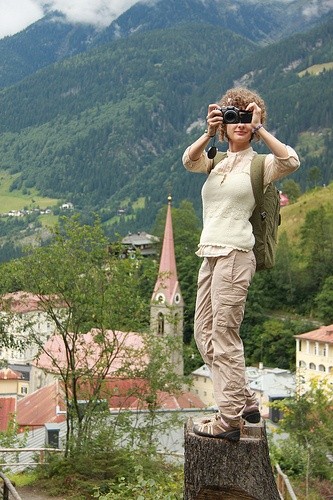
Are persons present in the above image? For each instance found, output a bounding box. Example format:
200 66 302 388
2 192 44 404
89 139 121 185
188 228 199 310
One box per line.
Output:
180 87 300 442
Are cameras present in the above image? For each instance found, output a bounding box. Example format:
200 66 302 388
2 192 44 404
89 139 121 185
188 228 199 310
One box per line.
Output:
218 106 253 124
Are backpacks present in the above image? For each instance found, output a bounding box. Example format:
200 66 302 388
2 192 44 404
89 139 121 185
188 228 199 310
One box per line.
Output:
209 152 281 271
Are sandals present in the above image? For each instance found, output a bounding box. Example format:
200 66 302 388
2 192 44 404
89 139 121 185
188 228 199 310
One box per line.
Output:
214 402 260 423
192 413 240 441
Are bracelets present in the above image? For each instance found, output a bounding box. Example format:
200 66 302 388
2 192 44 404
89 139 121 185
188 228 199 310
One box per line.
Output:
254 125 261 132
